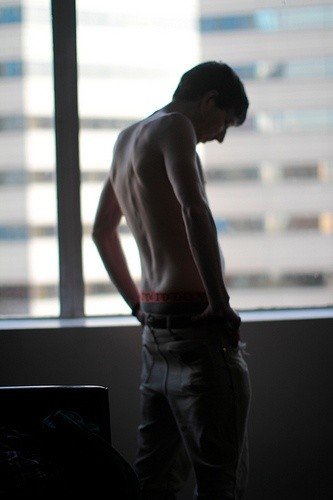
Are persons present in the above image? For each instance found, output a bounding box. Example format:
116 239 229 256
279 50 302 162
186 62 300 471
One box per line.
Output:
91 60 253 500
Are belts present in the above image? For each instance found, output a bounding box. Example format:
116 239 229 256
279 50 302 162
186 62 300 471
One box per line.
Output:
144 318 213 329
